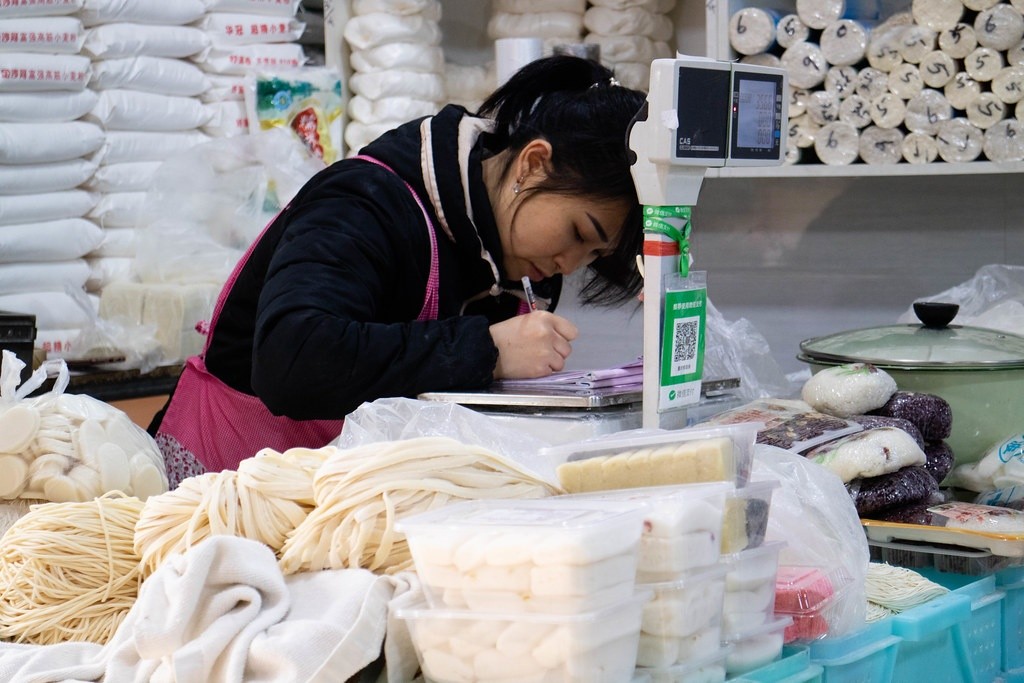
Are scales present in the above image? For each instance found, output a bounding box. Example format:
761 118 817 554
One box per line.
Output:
415 56 788 440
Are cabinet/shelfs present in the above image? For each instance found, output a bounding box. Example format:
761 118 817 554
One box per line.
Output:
318 1 1024 193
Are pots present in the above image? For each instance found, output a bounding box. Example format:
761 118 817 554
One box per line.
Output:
797 301 1023 478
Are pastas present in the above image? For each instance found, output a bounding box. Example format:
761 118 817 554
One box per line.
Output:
0 436 572 658
867 561 951 622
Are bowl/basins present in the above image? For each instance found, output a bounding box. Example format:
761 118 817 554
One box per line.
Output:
392 421 797 681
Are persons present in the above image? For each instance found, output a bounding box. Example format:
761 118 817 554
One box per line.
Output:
147 55 645 490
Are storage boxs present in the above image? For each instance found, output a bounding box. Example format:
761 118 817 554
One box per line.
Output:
722 562 1024 683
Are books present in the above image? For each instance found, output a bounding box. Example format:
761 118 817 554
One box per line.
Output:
498 356 643 388
